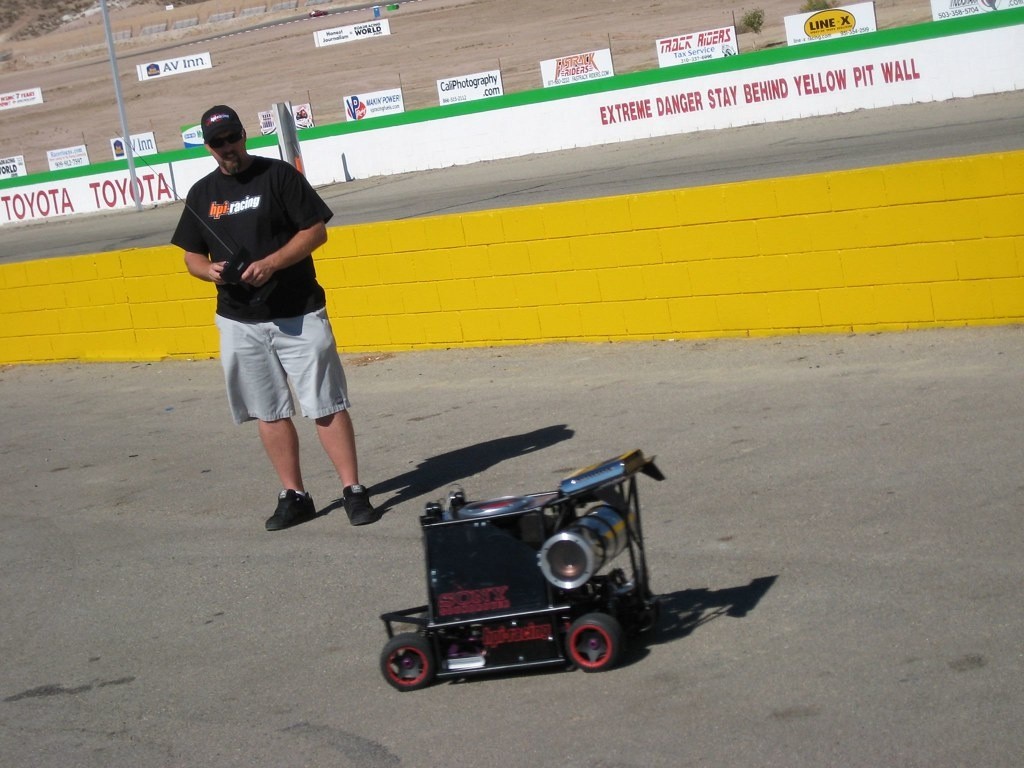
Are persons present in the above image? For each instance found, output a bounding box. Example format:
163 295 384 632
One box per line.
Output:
168 104 377 531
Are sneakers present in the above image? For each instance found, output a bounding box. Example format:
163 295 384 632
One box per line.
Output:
341 484 375 526
265 488 316 531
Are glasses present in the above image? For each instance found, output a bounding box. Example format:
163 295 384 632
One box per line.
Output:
208 131 242 149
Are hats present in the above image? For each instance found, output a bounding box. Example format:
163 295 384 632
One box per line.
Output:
200 105 242 140
